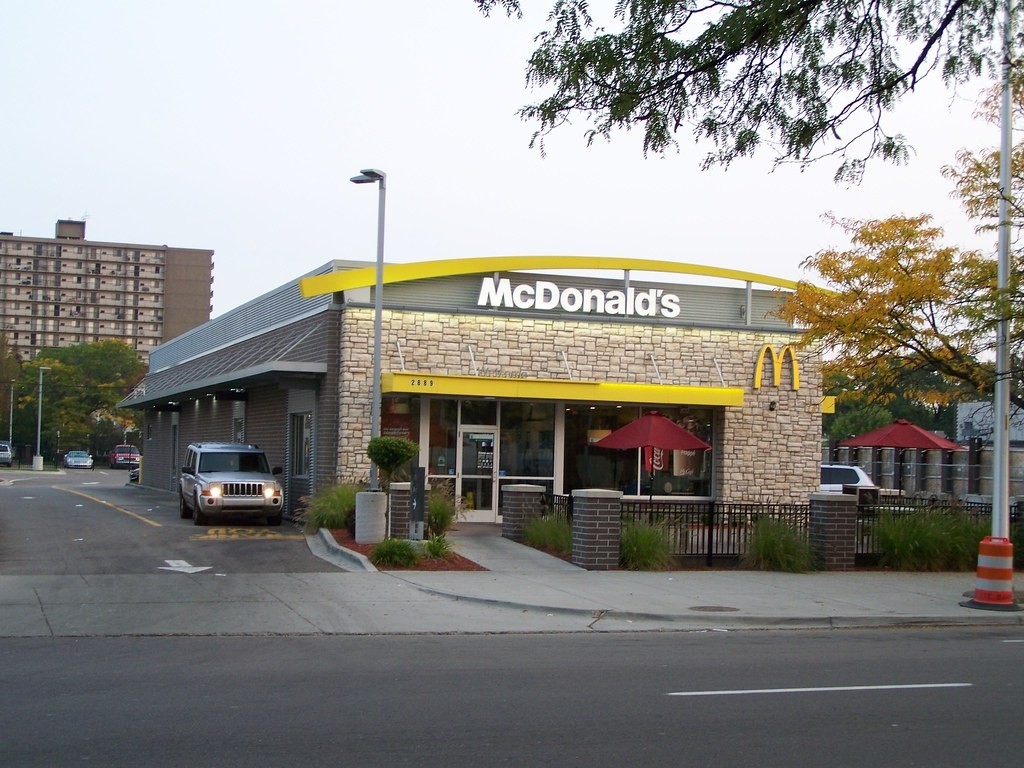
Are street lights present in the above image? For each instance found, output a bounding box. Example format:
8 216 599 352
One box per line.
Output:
9 378 15 447
347 168 394 547
32 367 52 471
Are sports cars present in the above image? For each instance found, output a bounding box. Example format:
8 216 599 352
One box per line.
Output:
63 451 93 469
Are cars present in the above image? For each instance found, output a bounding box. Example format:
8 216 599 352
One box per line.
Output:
108 445 140 468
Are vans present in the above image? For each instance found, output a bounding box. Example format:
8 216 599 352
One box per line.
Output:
0 444 13 468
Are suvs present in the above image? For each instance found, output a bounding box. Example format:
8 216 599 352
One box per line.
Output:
178 442 284 526
